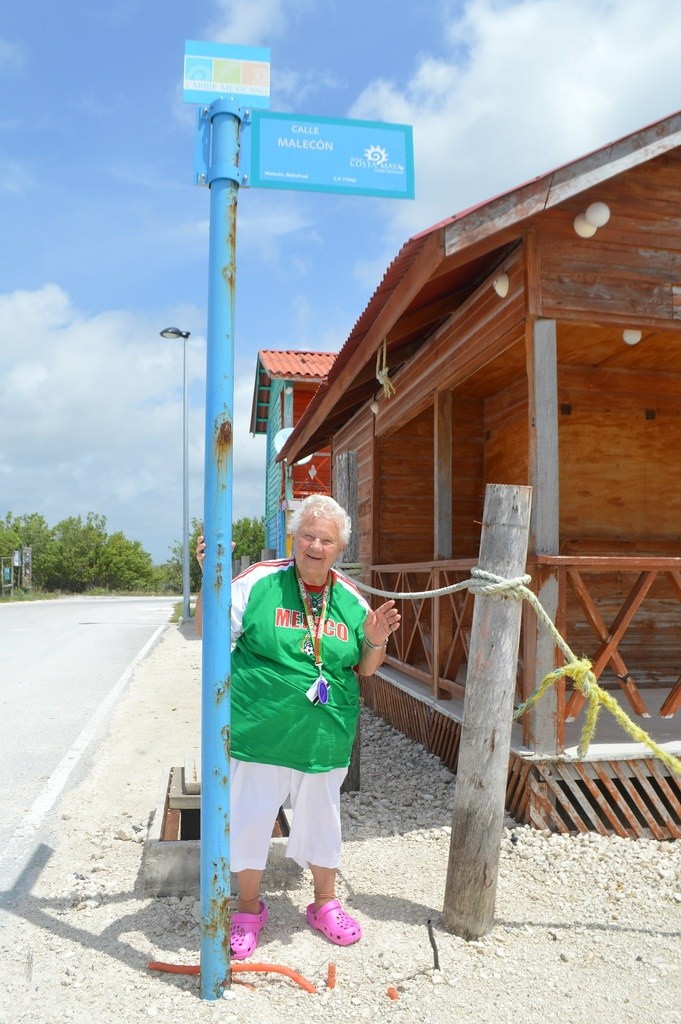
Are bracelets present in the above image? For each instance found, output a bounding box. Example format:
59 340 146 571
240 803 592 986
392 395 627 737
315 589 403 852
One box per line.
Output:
363 636 389 649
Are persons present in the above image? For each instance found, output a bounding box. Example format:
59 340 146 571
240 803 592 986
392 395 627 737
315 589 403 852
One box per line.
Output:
194 495 401 959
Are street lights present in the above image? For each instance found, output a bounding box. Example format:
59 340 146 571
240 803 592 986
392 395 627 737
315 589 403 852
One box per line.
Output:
158 327 194 627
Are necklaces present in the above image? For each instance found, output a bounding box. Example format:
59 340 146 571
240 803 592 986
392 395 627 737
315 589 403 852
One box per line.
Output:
297 580 324 615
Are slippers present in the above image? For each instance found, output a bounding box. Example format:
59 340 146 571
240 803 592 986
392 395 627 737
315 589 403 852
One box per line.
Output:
306 899 363 946
229 900 267 960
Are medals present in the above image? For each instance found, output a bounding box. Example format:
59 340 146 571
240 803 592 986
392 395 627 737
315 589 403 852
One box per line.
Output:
318 681 328 704
